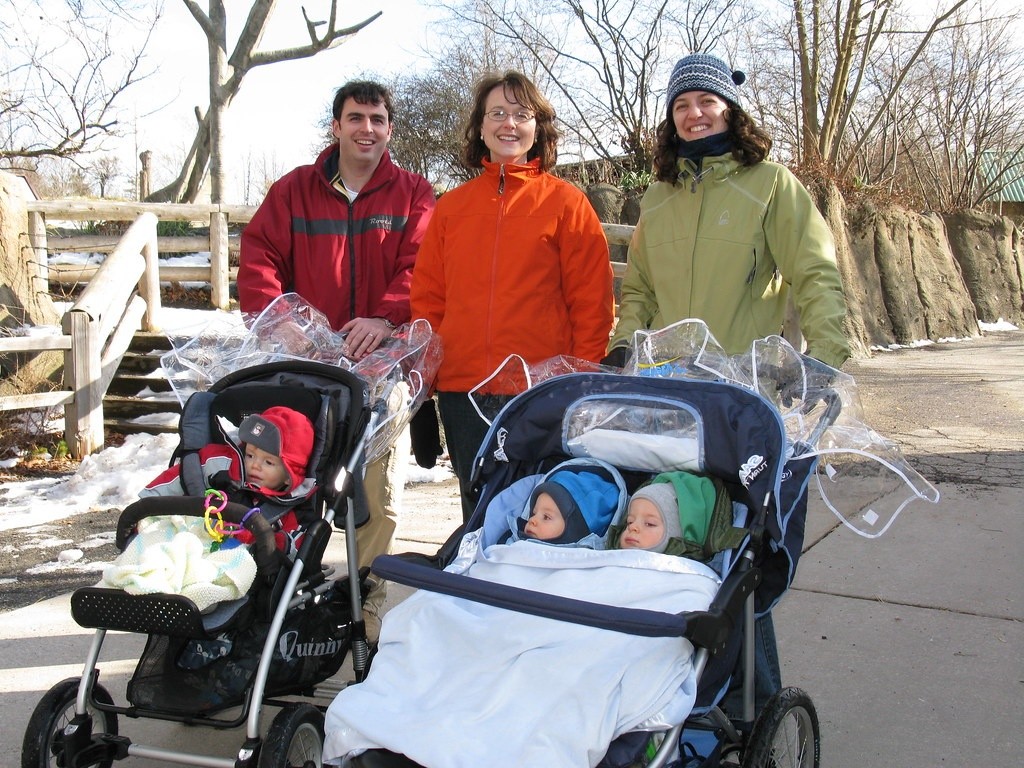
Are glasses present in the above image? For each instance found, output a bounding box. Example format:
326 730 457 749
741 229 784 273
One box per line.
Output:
484 110 536 122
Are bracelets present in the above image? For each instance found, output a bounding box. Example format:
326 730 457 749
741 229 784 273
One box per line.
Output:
383 319 396 328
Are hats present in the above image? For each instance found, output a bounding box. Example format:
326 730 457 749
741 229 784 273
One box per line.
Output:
238 405 314 495
665 55 746 110
621 482 683 553
529 470 619 538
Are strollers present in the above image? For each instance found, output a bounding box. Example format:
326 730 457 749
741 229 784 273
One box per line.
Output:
318 349 843 768
17 357 402 768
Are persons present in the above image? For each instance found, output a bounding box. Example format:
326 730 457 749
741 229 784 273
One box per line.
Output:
607 469 716 556
501 456 625 550
400 70 614 524
104 405 317 616
238 81 432 643
598 53 853 715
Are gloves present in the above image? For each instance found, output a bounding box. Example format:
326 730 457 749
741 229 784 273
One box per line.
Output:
407 399 444 469
777 355 835 414
600 347 632 373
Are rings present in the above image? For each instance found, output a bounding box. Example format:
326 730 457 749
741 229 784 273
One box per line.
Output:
368 332 374 337
